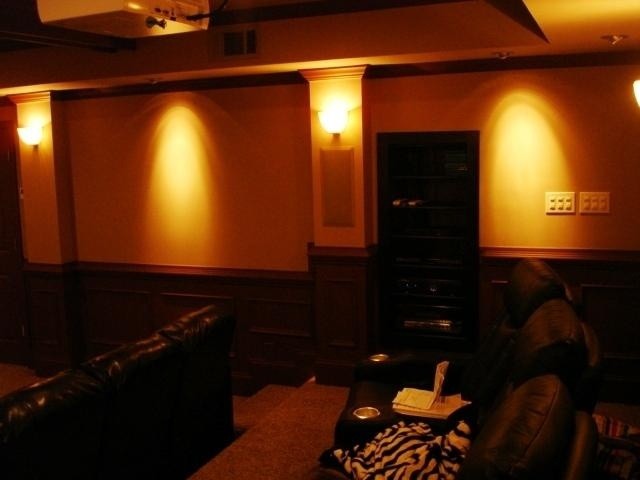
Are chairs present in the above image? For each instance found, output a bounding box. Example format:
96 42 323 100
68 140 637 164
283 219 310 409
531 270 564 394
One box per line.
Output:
0 302 238 478
309 258 606 480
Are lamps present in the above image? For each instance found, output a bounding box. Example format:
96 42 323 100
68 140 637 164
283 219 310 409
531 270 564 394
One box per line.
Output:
315 108 349 135
16 127 43 146
